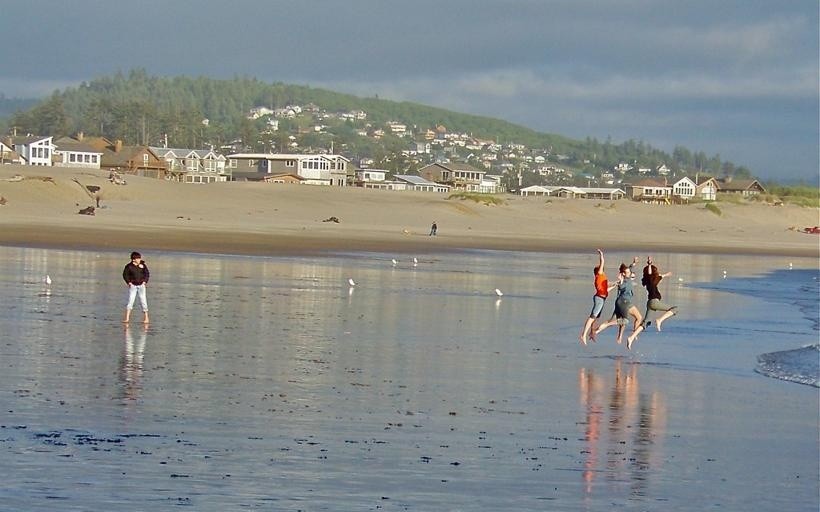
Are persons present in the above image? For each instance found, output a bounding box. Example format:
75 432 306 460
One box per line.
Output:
596 257 642 342
122 253 151 325
580 249 610 345
429 220 438 237
121 321 150 405
611 271 636 344
627 255 679 350
